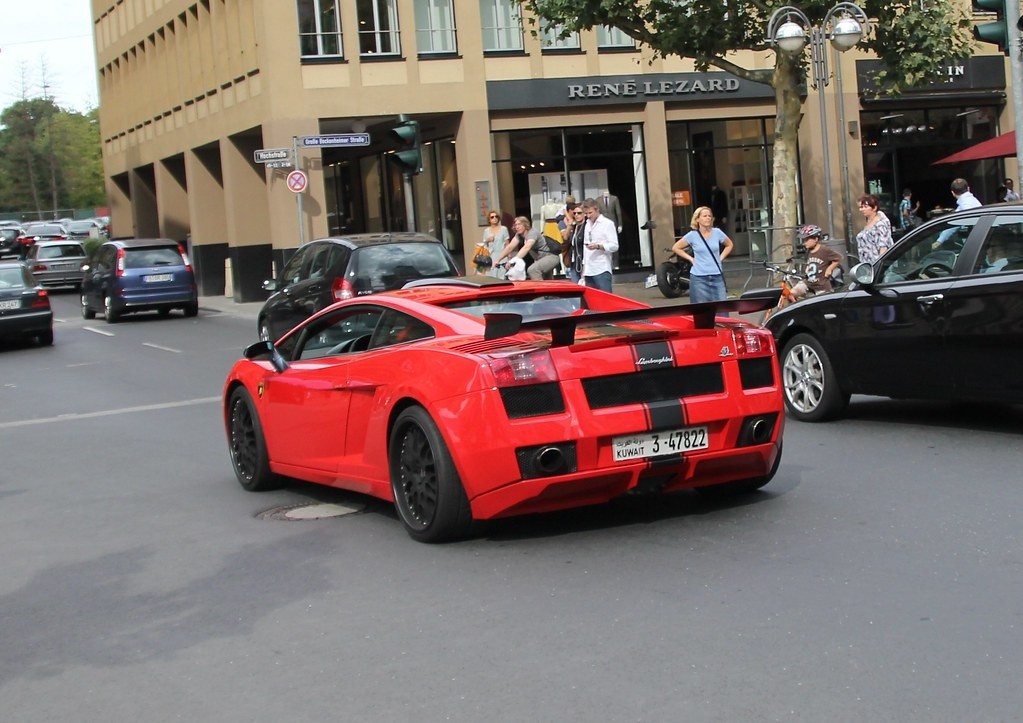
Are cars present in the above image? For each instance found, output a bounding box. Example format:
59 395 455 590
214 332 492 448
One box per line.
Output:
80 238 198 324
257 231 481 351
764 198 1023 425
23 239 92 292
0 216 111 260
0 258 54 348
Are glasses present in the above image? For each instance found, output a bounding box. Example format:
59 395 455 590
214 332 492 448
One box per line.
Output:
490 215 499 218
589 232 592 242
573 212 584 215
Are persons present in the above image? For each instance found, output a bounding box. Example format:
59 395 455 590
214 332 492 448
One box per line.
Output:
496 216 559 281
707 185 728 228
672 206 733 303
484 211 511 280
979 233 1009 273
540 199 566 275
993 179 1020 204
596 191 622 269
932 178 983 249
791 225 841 297
558 196 620 294
900 189 920 265
856 194 894 264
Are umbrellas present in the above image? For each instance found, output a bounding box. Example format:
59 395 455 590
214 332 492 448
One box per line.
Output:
932 130 1017 166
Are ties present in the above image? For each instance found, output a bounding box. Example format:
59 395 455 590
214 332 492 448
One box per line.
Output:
605 197 608 208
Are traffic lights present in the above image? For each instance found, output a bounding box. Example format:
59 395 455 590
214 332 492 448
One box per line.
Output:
971 0 1010 57
387 120 424 178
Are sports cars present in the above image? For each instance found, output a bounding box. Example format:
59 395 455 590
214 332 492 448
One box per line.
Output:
222 274 787 546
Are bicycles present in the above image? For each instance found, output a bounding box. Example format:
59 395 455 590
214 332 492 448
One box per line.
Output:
761 262 845 325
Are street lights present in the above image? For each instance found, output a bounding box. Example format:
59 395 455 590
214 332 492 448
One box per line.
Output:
767 2 872 269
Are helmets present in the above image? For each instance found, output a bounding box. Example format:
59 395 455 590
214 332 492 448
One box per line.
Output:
795 225 821 238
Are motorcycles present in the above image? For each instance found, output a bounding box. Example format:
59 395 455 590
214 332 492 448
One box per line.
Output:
656 235 694 298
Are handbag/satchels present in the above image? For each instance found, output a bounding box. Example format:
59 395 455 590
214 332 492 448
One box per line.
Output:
578 278 585 286
469 241 492 274
504 257 526 281
473 243 492 265
559 240 571 267
543 235 562 255
